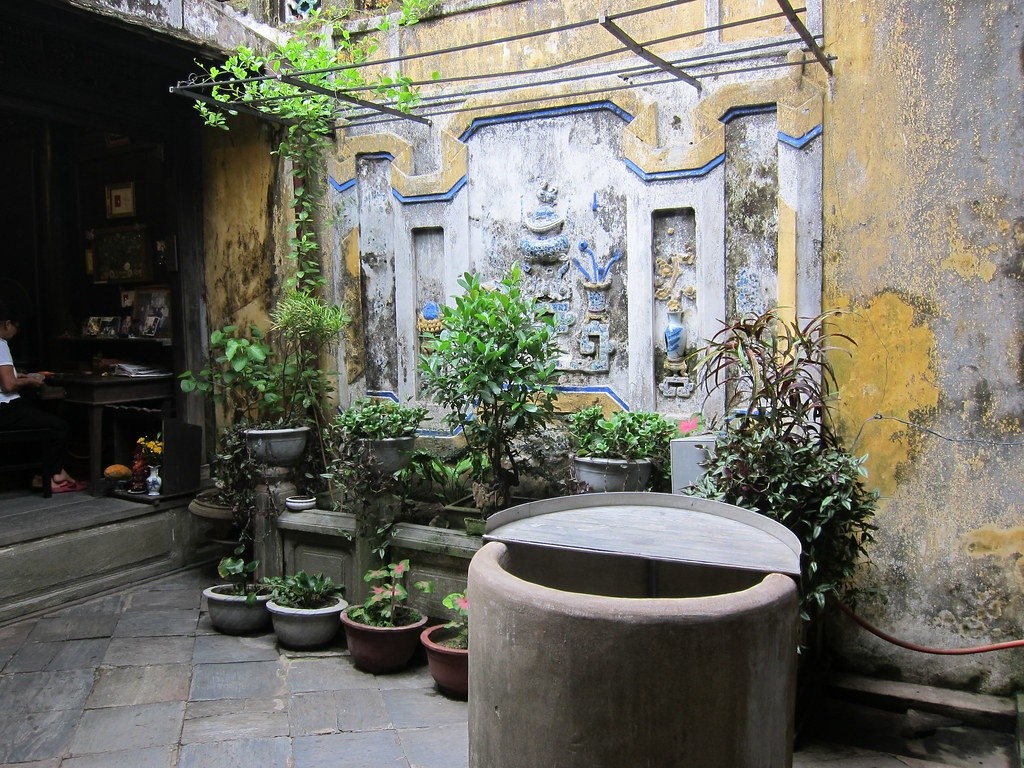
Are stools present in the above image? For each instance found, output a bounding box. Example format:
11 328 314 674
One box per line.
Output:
0 430 51 497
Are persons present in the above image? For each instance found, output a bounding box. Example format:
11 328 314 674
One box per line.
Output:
0 300 90 492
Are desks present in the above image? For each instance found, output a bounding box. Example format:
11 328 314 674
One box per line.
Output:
42 371 173 497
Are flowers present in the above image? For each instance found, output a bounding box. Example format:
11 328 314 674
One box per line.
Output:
138 433 165 466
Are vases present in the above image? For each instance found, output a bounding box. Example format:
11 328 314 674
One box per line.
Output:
286 494 317 512
145 464 162 496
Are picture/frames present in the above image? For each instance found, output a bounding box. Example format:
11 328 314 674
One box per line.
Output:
103 132 132 149
106 181 138 219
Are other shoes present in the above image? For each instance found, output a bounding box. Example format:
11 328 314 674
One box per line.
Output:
51 477 88 493
32 474 43 487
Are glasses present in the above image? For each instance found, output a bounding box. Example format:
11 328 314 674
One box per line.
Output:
12 322 20 334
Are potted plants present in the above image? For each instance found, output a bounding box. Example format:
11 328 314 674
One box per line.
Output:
246 567 351 651
564 403 669 495
186 422 257 552
412 258 547 528
202 544 270 633
328 392 432 475
339 559 436 675
181 328 330 467
419 585 471 697
